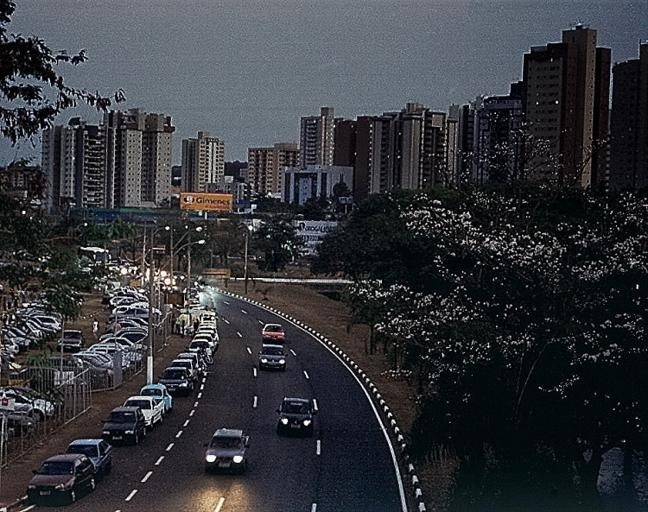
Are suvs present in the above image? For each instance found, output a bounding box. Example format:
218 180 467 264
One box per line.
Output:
101 405 148 444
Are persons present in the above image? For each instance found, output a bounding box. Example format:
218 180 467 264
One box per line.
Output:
27 374 39 390
91 318 100 338
170 313 176 333
175 314 181 333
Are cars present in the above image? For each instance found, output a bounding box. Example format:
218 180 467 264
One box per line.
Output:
261 322 288 344
276 396 318 438
258 343 289 371
203 425 253 477
26 453 98 504
1 386 65 437
1 245 219 393
64 438 113 482
139 385 173 411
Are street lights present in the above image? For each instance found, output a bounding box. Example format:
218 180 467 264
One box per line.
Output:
145 221 206 384
244 224 256 294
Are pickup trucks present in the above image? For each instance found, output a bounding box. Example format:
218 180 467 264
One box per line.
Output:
122 395 165 428
